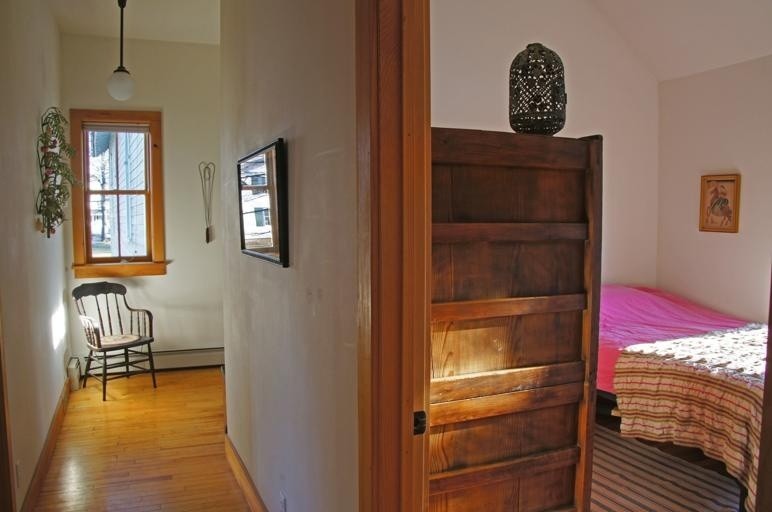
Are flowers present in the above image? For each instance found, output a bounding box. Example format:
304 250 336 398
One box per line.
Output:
34 106 83 237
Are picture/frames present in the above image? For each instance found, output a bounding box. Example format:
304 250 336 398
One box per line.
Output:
700 174 742 234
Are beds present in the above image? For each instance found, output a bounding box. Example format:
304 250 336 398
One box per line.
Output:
597 283 770 491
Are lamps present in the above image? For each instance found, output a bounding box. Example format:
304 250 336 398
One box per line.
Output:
107 0 135 99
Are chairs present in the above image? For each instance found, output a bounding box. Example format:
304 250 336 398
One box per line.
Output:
71 281 157 401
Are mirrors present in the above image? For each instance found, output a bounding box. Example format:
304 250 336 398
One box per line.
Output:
236 138 291 268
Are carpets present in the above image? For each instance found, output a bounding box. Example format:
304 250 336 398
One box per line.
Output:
591 423 740 512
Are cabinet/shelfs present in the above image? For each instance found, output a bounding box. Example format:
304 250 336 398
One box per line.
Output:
430 127 603 510
